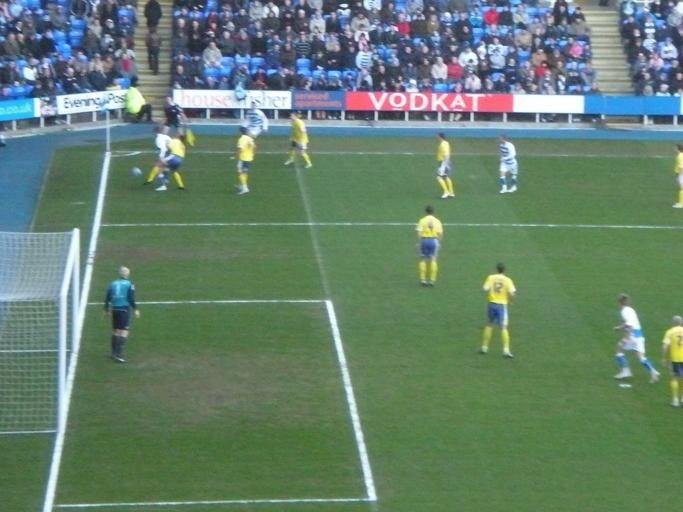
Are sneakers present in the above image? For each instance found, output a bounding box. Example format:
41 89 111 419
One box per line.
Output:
284 159 312 168
480 346 514 359
671 400 679 406
614 369 632 379
500 185 517 193
650 372 660 382
143 181 186 192
440 193 455 198
421 281 434 286
673 203 683 208
234 184 249 194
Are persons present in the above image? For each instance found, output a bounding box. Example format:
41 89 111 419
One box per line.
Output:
234 127 256 196
416 205 443 287
620 1 682 124
144 1 163 74
229 102 268 160
143 96 188 191
480 263 516 358
498 133 518 194
672 143 683 208
284 112 313 169
436 133 456 199
659 315 683 408
0 1 139 122
170 1 604 121
103 266 139 363
613 294 661 385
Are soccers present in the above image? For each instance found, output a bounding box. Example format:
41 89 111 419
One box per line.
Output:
132 167 141 176
